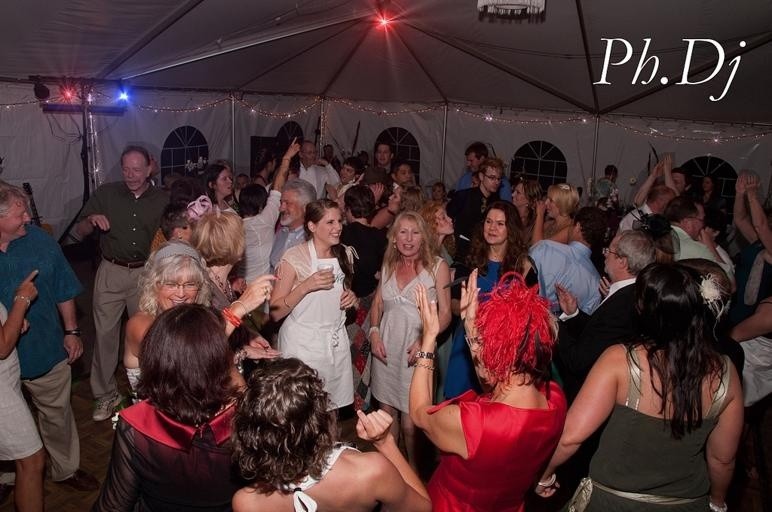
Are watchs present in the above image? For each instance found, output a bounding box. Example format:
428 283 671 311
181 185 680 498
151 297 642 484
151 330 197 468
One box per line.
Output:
412 350 436 360
64 328 83 336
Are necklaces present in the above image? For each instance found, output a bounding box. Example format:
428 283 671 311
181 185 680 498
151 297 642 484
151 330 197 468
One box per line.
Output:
214 273 233 301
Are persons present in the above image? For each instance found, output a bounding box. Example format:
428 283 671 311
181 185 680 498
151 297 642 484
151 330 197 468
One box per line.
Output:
230 358 432 512
160 200 192 242
269 198 358 442
427 182 448 208
730 168 772 310
387 182 421 215
532 183 579 244
363 142 395 202
149 154 161 187
1 180 99 493
447 157 503 263
663 194 737 294
297 140 341 199
634 213 680 261
695 173 727 232
442 199 536 401
88 301 246 512
553 230 657 404
75 145 171 421
235 173 249 202
150 176 207 252
325 156 362 225
370 183 422 232
122 240 245 405
0 270 46 512
633 154 687 208
408 267 568 512
614 185 675 234
190 211 277 359
250 146 277 188
528 205 608 316
454 141 512 201
204 163 238 215
322 145 341 175
418 199 454 266
232 137 300 314
511 180 542 232
728 295 772 410
269 178 317 349
340 184 389 325
367 211 453 472
390 162 413 185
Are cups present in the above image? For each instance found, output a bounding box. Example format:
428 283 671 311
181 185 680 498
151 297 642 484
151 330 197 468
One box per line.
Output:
317 263 334 275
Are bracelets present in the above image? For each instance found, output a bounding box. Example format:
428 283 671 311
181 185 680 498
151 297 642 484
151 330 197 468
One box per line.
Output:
708 496 728 512
463 335 479 346
229 299 247 311
368 326 379 337
12 295 31 307
539 473 557 487
221 307 242 329
413 362 436 371
283 298 292 309
534 260 743 512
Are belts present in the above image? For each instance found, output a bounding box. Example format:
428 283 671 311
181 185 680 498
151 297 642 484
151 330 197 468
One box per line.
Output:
101 255 145 268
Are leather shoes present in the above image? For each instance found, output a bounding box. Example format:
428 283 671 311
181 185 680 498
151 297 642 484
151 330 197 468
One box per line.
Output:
58 470 100 491
1 483 13 504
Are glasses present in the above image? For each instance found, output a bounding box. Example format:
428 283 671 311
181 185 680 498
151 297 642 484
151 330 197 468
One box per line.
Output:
122 168 150 173
601 247 624 260
687 214 704 223
303 150 318 154
483 172 503 180
156 279 201 293
